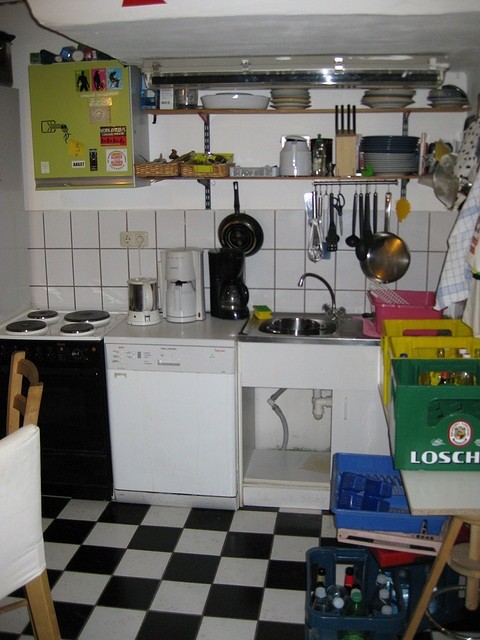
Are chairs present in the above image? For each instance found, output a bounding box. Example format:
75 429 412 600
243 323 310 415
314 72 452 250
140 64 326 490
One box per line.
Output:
5 350 43 432
1 425 61 640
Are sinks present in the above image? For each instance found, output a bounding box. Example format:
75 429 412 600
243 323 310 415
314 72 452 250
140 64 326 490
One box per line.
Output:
266 317 337 337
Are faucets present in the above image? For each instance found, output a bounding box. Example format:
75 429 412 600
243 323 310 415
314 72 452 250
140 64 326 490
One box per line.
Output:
298 272 338 318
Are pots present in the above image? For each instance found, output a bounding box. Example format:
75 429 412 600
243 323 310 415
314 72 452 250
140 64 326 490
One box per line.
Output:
359 192 411 286
216 180 265 257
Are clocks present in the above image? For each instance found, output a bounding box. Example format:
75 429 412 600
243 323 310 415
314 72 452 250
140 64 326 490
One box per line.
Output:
71 50 85 62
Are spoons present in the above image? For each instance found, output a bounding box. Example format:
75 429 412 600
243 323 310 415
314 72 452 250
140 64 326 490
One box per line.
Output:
345 192 375 263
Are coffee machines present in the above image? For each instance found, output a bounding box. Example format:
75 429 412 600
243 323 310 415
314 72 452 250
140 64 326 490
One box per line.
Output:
209 248 251 322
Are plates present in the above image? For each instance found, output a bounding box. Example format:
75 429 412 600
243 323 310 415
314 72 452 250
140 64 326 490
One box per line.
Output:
358 133 422 177
428 83 471 112
200 88 271 116
357 85 424 111
266 86 319 113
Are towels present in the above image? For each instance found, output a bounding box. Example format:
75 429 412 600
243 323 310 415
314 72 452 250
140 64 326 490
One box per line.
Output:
461 214 480 339
432 167 480 320
453 118 480 184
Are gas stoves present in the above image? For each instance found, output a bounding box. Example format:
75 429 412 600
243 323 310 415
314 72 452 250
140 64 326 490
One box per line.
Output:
3 302 112 339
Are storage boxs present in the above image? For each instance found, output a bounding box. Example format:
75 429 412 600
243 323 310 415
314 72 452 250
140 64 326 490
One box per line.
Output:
367 288 442 336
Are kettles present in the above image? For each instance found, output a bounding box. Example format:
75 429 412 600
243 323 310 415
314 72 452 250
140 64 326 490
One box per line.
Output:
164 249 207 324
128 278 160 327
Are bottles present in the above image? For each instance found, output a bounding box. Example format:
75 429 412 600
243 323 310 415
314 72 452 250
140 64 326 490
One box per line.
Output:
381 605 394 615
394 570 409 610
438 372 454 383
60 47 71 60
343 567 362 598
311 586 330 615
457 345 473 386
436 348 446 358
373 589 398 615
315 567 328 589
343 593 367 640
327 584 345 610
312 146 327 177
400 352 408 359
426 586 438 617
373 573 388 590
